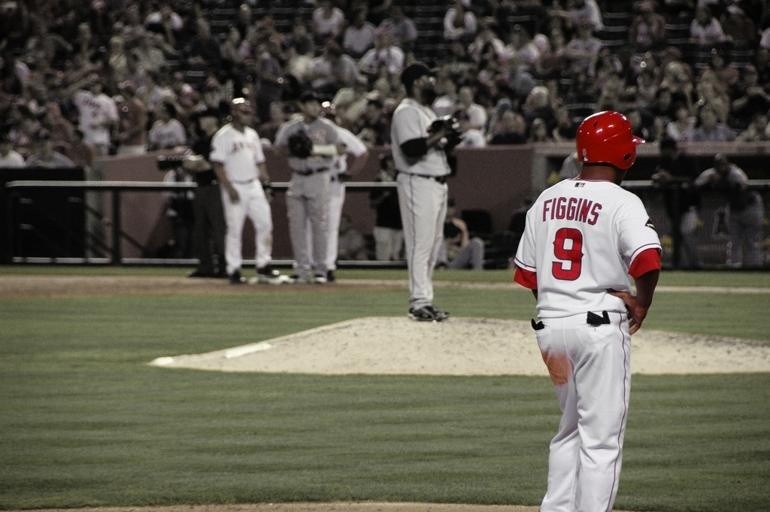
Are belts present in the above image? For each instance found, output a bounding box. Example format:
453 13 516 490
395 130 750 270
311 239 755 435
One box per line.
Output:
398 169 448 183
292 164 328 176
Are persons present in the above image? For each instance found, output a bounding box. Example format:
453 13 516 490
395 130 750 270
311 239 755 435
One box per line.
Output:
207 88 368 284
511 109 664 512
2 0 770 271
388 59 466 322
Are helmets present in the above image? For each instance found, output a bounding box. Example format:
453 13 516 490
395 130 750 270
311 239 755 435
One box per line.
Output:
577 112 647 170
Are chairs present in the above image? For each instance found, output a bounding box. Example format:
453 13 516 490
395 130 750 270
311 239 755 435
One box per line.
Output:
460 209 508 270
1 0 769 161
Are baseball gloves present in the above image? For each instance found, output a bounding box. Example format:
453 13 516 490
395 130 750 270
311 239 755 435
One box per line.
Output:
288 129 312 159
428 117 462 150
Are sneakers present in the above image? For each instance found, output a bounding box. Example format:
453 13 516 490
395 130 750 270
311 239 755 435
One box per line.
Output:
407 305 450 322
229 265 335 284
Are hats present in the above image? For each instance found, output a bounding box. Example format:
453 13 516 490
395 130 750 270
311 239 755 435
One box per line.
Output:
298 90 320 104
400 63 441 83
